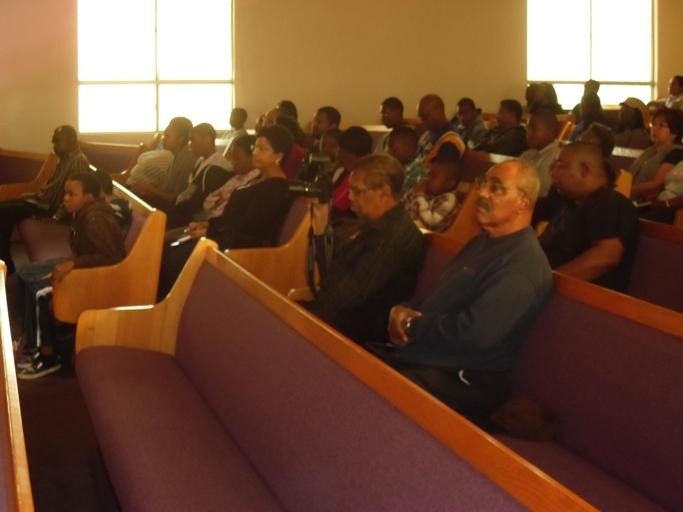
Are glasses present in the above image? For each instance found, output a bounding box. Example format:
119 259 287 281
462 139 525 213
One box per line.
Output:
475 176 504 193
250 144 274 153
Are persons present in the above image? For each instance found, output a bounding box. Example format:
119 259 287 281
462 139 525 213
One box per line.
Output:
15 171 126 381
0 125 90 275
7 169 133 354
125 69 683 338
359 158 554 422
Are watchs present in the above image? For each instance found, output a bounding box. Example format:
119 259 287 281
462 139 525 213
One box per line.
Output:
401 315 413 336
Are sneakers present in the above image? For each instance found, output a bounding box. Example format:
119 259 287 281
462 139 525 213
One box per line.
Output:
18 353 41 368
18 356 61 379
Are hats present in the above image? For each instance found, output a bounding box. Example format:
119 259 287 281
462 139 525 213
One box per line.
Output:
619 97 645 110
